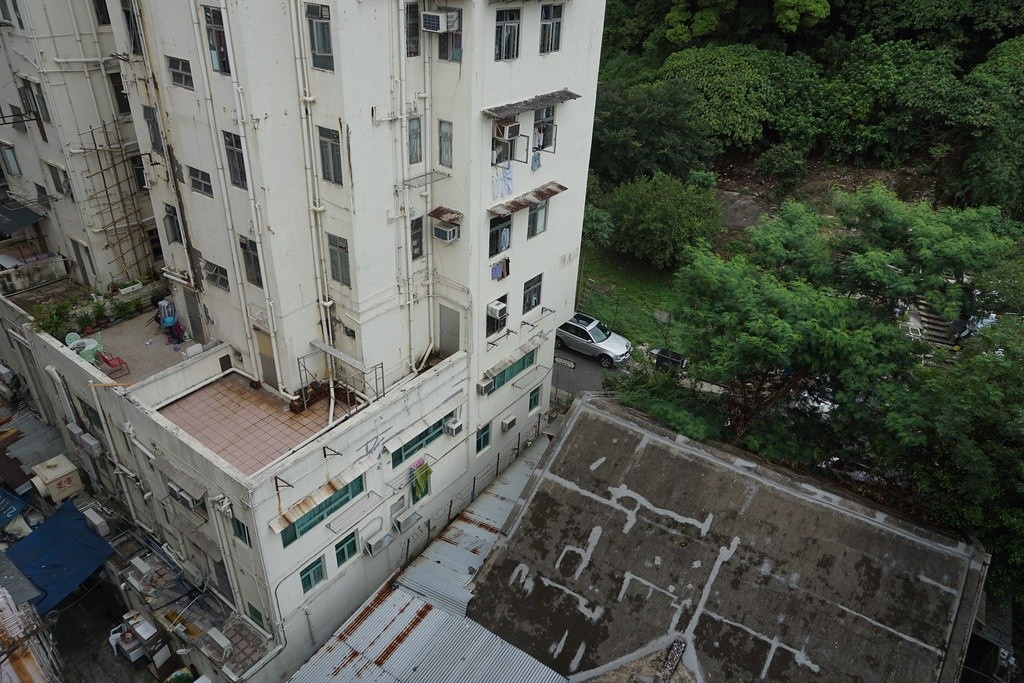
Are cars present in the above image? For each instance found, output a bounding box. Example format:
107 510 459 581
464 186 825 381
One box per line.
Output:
556 312 634 369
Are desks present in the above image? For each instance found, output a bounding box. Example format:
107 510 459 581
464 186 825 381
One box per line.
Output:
68 339 99 362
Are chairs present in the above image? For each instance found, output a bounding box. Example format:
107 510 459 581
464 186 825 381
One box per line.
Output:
80 349 101 369
65 332 81 346
97 350 130 379
108 624 126 656
145 310 161 335
93 331 104 355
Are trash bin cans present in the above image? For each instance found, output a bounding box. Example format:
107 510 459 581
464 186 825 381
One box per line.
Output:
656 347 684 370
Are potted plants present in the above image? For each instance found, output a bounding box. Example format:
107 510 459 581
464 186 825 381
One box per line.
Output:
29 270 170 343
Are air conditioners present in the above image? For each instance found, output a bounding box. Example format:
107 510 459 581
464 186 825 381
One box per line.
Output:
367 529 390 557
83 508 110 537
207 627 232 659
488 300 507 318
396 507 417 534
168 481 182 500
495 120 520 140
432 222 459 242
421 10 458 33
446 419 463 436
502 416 516 431
179 491 203 509
476 377 494 396
0 381 13 400
0 588 22 637
80 433 102 457
130 556 152 582
0 364 13 384
66 422 84 445
182 561 203 586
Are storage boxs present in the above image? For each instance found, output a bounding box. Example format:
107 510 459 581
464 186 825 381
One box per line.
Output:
27 511 45 526
